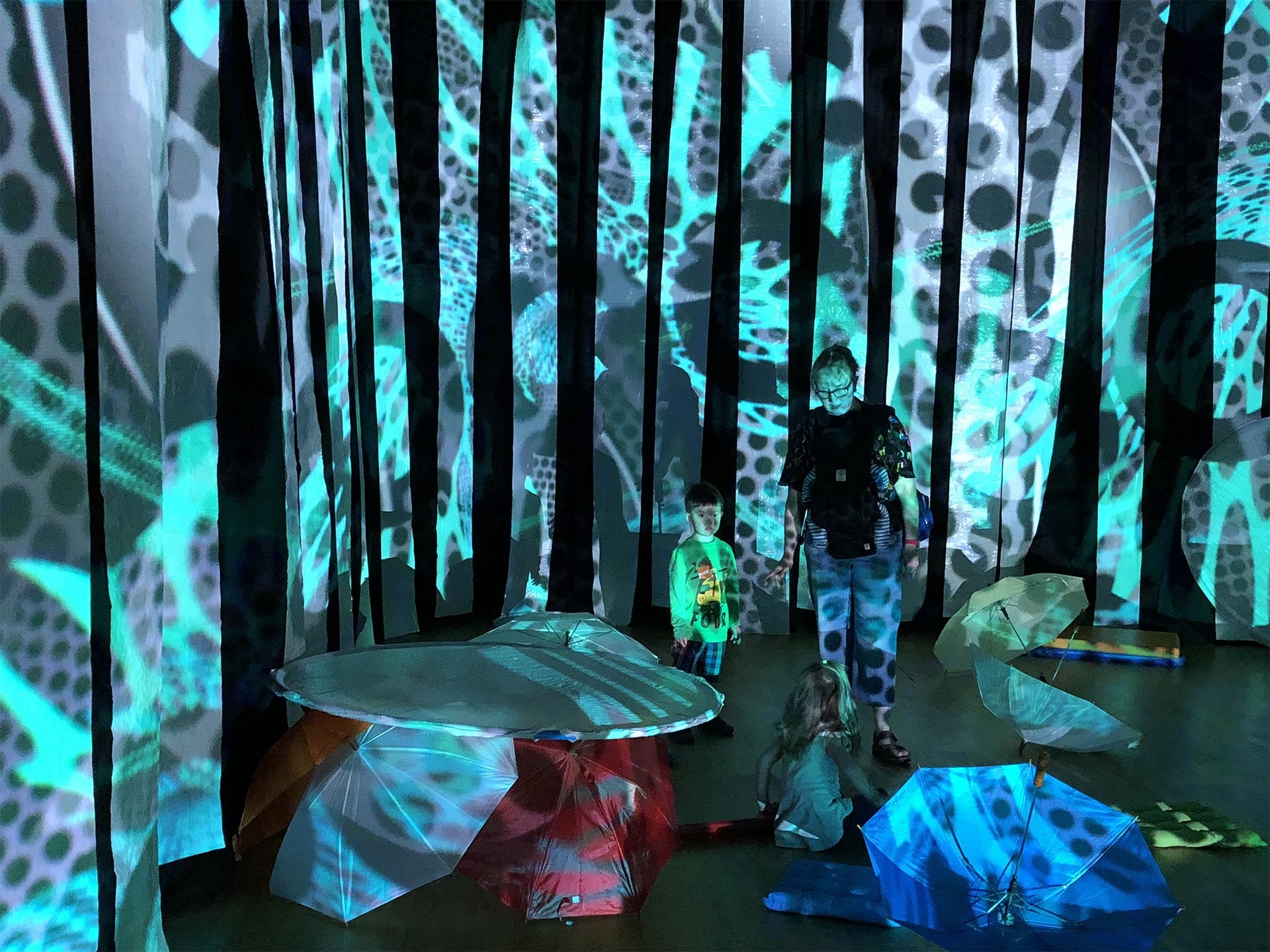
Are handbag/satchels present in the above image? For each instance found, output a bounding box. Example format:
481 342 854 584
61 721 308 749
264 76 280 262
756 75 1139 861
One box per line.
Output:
916 488 934 541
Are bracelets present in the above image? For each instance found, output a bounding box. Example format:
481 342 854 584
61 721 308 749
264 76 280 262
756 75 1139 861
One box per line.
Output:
903 539 921 547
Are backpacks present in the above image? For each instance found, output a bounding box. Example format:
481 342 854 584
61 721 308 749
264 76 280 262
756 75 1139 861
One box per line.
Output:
804 407 894 559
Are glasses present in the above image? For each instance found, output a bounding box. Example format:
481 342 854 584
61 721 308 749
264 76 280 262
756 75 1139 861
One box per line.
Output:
811 382 853 400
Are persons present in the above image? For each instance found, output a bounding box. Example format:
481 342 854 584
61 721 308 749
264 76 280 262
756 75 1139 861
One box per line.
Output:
765 345 922 767
756 661 893 857
660 484 742 745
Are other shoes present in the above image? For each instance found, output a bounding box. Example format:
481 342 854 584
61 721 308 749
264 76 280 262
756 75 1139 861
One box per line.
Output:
667 729 695 745
872 731 912 766
679 821 720 846
703 717 735 737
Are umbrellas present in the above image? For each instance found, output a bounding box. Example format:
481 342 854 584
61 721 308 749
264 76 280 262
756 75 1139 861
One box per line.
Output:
229 603 726 925
856 751 1191 952
933 572 1090 685
969 610 1144 756
